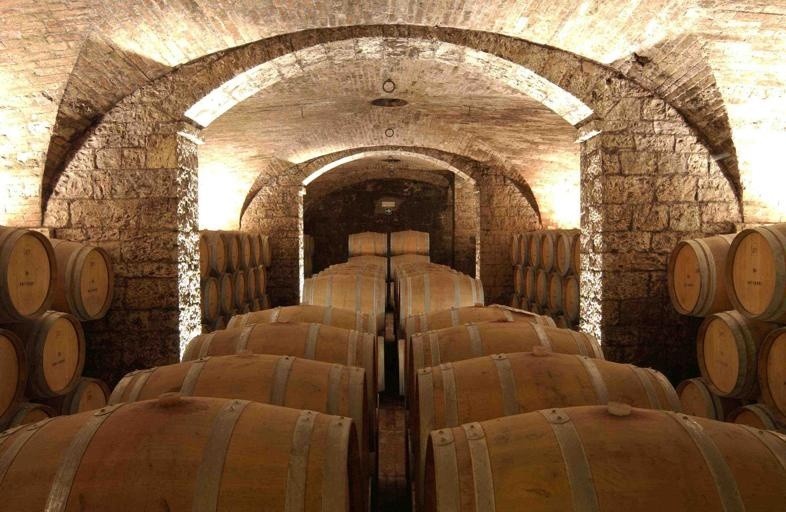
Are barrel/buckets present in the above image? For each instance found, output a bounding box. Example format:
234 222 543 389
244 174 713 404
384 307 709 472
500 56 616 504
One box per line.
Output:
199 228 271 335
1 225 115 433
2 303 376 510
666 223 785 431
347 231 430 310
301 263 485 409
508 228 581 332
403 304 786 512
